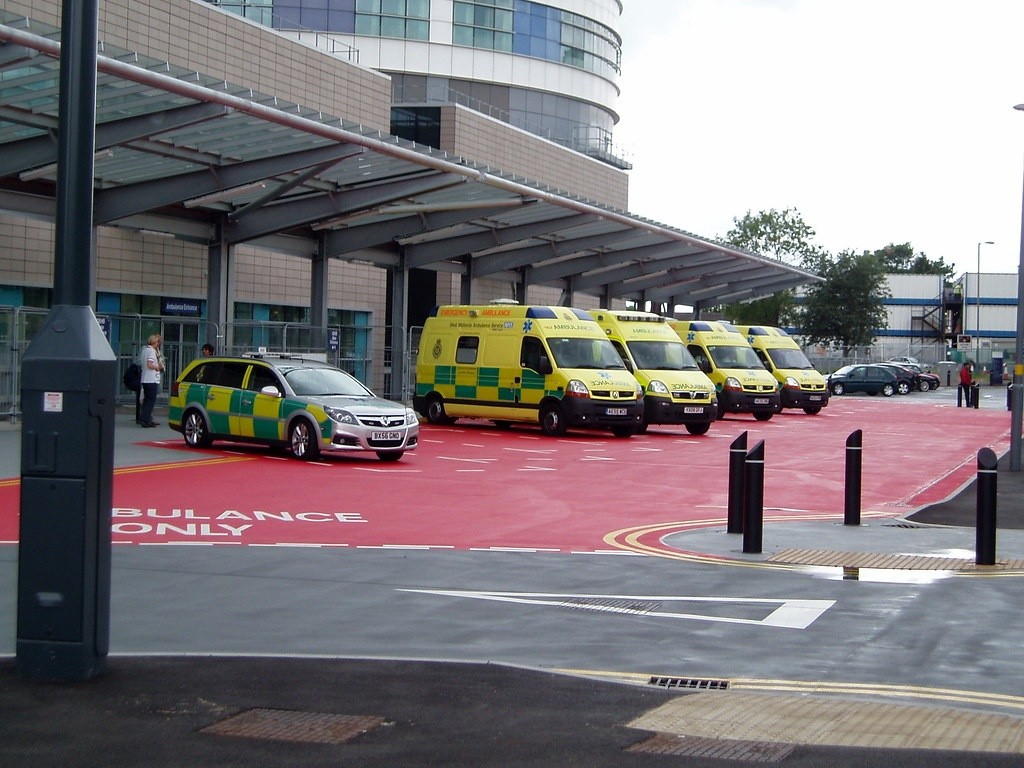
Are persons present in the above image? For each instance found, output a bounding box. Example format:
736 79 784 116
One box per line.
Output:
202 344 214 357
960 363 972 408
136 333 166 428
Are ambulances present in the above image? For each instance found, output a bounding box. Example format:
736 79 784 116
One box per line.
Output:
715 318 833 416
583 306 718 435
663 316 780 421
412 297 646 438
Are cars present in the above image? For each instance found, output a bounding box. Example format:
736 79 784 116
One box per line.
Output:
856 355 941 395
167 349 420 464
821 364 898 397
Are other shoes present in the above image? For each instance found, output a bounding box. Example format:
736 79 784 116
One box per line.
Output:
137 417 161 429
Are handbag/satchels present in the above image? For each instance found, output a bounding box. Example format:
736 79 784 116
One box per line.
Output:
123 363 142 393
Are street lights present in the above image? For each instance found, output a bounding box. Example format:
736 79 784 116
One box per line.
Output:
975 241 995 369
